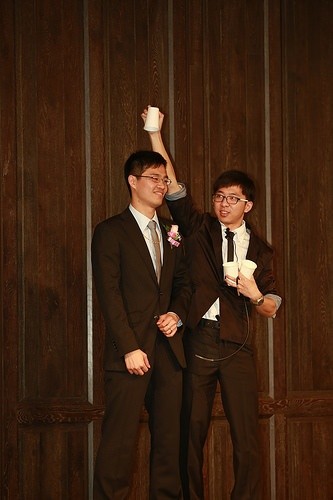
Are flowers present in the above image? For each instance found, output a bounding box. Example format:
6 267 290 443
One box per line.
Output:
164 224 182 249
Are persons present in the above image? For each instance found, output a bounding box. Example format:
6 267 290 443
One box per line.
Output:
141 104 283 500
91 150 191 500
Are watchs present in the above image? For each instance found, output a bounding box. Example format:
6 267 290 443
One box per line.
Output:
250 294 264 306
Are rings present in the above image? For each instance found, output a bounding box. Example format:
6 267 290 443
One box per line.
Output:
170 329 173 333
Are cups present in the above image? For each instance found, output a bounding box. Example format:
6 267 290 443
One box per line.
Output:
143 107 160 132
222 261 238 281
240 259 258 280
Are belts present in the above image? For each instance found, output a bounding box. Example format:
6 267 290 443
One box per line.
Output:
200 319 220 329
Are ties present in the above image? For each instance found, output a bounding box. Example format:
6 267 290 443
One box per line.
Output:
225 228 235 261
147 220 161 285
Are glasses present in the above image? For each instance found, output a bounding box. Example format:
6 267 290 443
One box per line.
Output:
212 193 248 205
133 175 171 185
